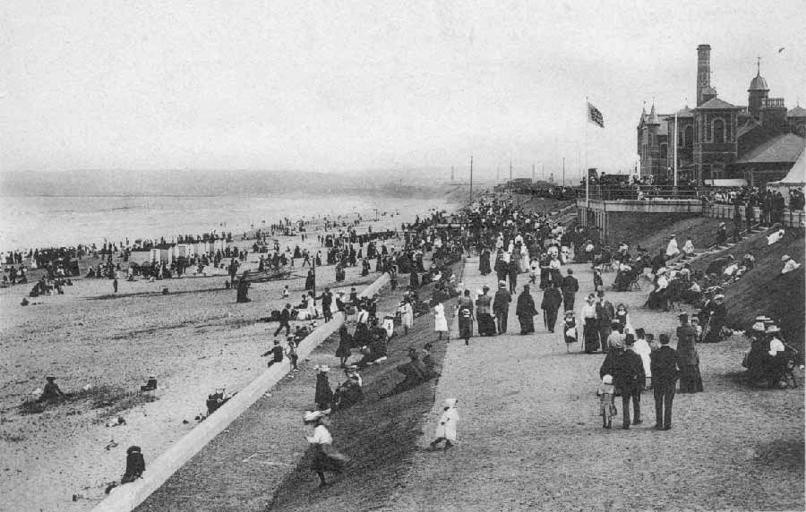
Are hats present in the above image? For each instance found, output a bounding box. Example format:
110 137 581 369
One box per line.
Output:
714 294 726 301
624 333 637 344
598 290 605 297
678 311 690 319
584 293 601 303
462 309 470 315
303 408 331 423
603 374 613 384
655 268 670 276
320 365 331 372
781 254 791 262
615 302 628 310
610 318 621 324
752 315 781 335
541 253 548 259
564 310 576 318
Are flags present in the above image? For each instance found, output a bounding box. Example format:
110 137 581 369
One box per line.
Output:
588 102 603 128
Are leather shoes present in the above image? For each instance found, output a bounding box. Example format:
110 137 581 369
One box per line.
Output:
664 424 673 430
633 419 644 425
319 481 326 488
